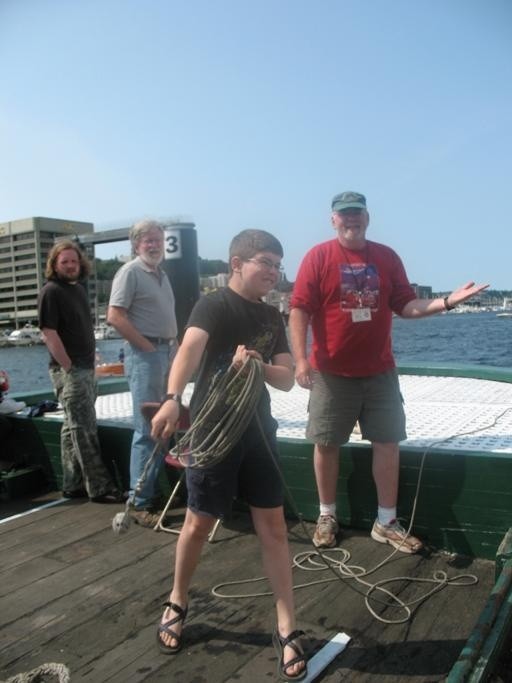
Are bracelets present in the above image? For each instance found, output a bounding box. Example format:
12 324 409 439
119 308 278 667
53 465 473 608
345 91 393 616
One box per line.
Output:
443 297 455 311
159 394 181 407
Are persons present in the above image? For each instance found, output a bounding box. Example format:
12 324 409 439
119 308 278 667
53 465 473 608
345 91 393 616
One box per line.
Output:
119 348 125 363
106 218 183 530
36 239 126 503
149 229 309 682
287 191 491 555
94 347 102 365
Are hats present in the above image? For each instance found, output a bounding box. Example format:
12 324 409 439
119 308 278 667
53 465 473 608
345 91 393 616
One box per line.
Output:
331 189 367 211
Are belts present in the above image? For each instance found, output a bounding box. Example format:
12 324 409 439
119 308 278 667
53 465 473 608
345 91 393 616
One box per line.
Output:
141 334 178 344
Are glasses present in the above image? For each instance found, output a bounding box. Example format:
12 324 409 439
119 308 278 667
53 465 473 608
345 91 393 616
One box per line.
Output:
245 255 282 269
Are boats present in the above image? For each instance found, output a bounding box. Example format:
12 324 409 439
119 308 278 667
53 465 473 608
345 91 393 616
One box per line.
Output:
2 365 512 681
97 362 125 377
0 319 121 347
497 311 512 317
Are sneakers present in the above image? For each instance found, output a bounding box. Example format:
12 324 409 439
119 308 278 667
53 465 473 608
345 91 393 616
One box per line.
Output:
128 507 167 528
312 513 340 548
370 516 424 554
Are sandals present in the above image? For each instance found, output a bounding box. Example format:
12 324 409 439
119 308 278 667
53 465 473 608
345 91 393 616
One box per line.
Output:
155 599 188 655
271 627 308 682
61 487 87 498
91 487 129 503
